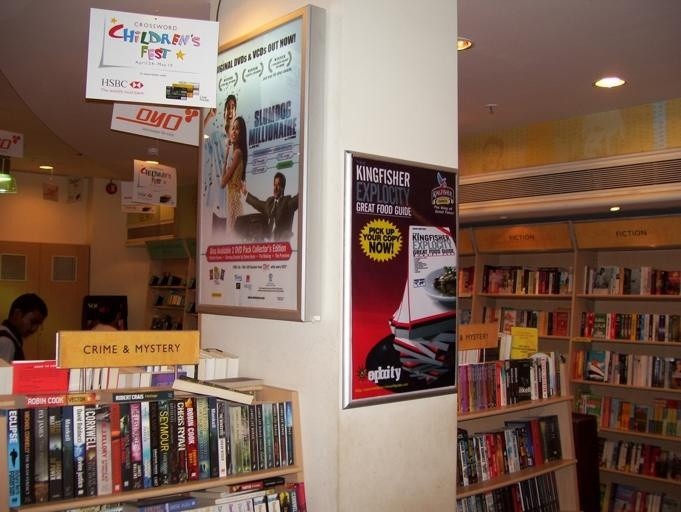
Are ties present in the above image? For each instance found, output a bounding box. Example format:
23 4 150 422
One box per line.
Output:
265 201 277 240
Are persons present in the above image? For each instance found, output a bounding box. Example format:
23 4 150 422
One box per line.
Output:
236 172 299 241
201 93 239 234
217 116 249 235
0 291 50 364
87 303 118 332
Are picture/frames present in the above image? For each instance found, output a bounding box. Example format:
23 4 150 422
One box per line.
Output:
345 150 459 409
195 6 310 322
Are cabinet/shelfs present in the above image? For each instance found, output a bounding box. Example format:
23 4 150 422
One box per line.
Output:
145 259 197 330
0 386 306 512
456 245 681 512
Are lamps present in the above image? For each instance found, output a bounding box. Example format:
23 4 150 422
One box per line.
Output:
1 156 17 194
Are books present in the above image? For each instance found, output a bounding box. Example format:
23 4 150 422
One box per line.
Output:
459 265 573 365
6 375 307 512
576 312 681 343
574 350 680 390
1 347 240 397
457 352 567 415
457 415 564 512
575 394 681 438
574 265 681 296
596 432 681 511
